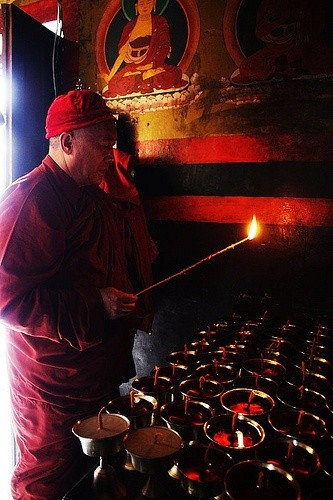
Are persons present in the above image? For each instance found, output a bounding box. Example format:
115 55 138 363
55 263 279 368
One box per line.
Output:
1 88 156 500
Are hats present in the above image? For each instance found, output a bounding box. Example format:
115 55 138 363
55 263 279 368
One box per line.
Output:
45 90 117 139
